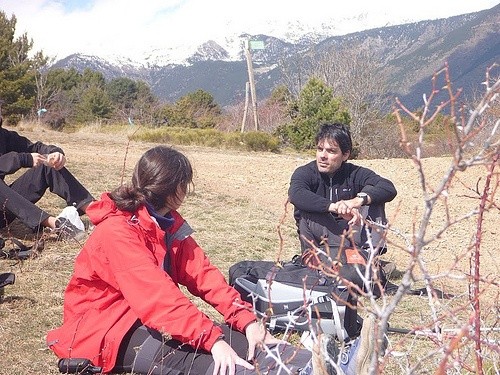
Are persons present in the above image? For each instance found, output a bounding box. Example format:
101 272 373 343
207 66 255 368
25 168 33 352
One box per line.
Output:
46 146 377 375
287 123 398 300
0 116 98 240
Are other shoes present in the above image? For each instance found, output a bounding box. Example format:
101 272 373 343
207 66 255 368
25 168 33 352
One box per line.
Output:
373 257 396 275
335 314 375 375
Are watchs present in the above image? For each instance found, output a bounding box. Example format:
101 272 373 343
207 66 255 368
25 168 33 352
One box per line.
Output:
356 193 368 207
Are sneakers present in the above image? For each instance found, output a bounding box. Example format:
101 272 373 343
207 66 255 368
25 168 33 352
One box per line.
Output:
55 218 85 242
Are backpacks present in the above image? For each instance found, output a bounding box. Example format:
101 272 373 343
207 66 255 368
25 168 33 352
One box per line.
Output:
229 253 358 339
294 244 387 297
